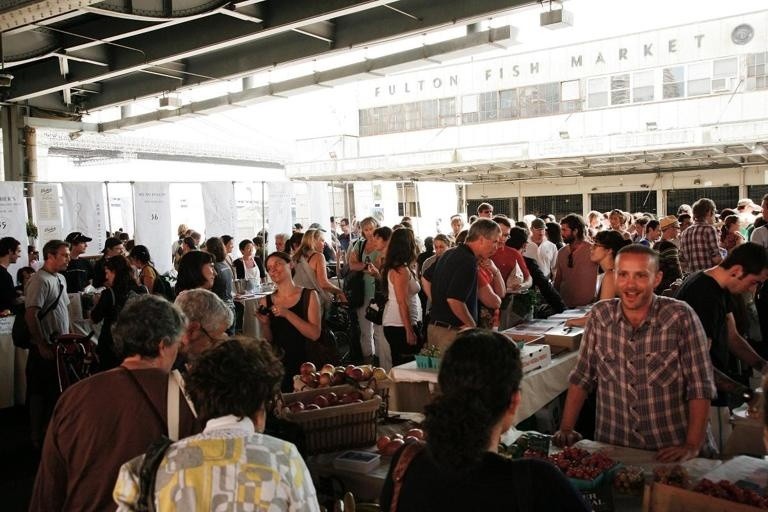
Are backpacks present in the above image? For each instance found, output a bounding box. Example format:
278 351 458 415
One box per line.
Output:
148 265 173 301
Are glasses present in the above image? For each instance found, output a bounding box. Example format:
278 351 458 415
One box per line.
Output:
568 253 574 267
591 243 609 249
201 328 225 348
504 234 512 240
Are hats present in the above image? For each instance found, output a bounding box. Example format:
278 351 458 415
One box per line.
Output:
738 199 755 208
532 219 548 229
101 237 123 253
676 204 692 221
66 232 92 243
660 214 682 231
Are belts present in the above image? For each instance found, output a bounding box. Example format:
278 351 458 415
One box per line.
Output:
426 320 460 330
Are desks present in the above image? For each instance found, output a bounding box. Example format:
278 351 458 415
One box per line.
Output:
304 406 767 511
382 346 578 430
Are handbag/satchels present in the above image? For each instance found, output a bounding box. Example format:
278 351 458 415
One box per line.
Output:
290 318 338 358
324 289 334 311
13 302 40 349
365 298 386 326
498 289 535 332
344 269 365 307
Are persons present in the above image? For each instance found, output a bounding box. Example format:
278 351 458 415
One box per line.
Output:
1 229 157 451
28 295 197 510
378 327 590 510
109 336 323 510
165 191 768 466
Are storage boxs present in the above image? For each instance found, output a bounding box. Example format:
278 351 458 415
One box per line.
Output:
518 343 553 376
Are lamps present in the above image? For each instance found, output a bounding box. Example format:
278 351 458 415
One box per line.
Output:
539 0 576 33
157 89 185 111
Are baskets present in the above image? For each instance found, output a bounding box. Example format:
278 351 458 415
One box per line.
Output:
293 366 393 424
272 384 382 455
334 450 382 474
557 461 623 511
414 353 442 372
